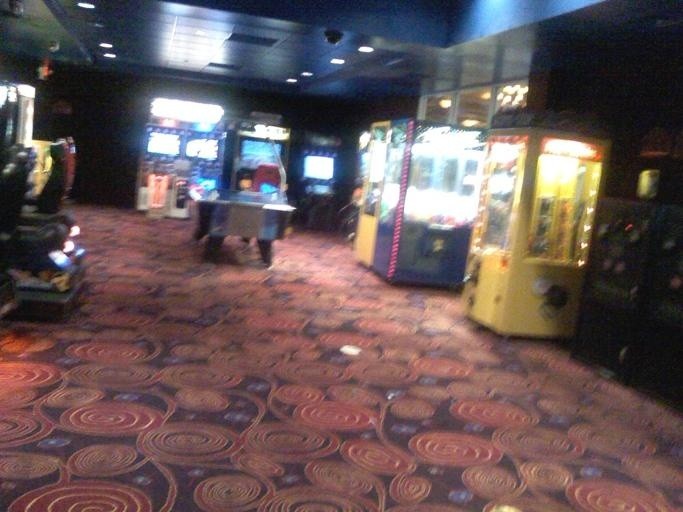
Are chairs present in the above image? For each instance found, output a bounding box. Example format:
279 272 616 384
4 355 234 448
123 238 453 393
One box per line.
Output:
2 135 79 267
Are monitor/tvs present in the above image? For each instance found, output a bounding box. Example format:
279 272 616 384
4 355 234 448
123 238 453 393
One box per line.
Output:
303 155 335 180
185 137 219 160
146 131 181 156
239 137 285 162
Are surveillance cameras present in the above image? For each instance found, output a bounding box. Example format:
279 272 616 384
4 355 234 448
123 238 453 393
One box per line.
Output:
321 29 344 44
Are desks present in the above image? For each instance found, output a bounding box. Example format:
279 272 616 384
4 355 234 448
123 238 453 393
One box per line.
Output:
194 199 298 267
